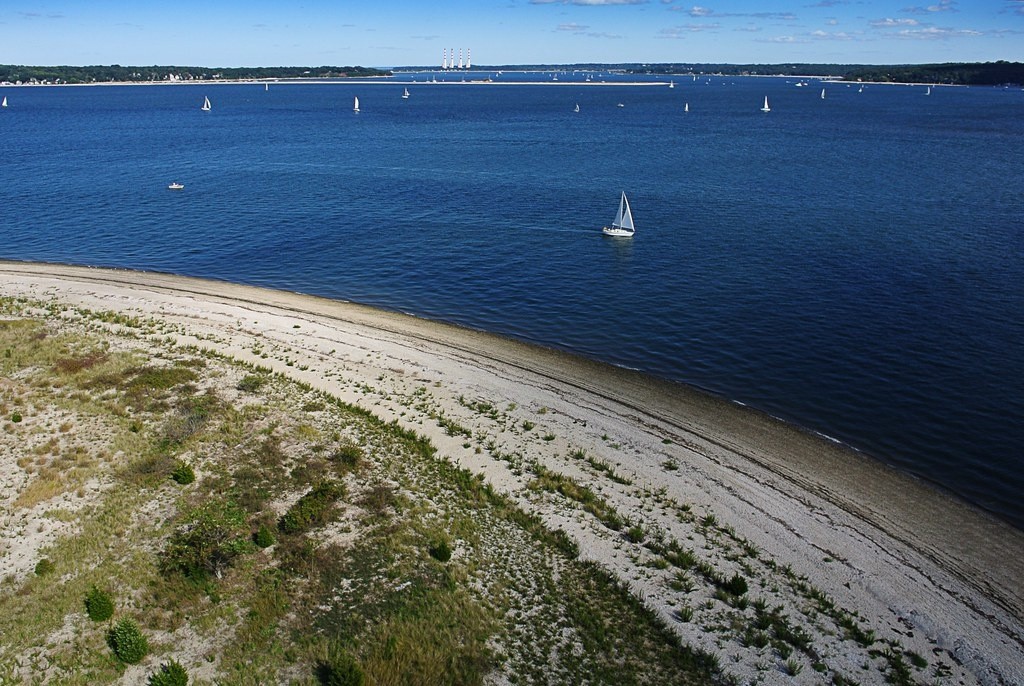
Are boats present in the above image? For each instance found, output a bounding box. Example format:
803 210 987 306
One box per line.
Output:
169 182 185 189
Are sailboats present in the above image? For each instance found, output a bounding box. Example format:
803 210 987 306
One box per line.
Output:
820 88 826 100
200 95 212 111
760 94 771 114
353 95 360 112
925 86 931 96
401 87 410 99
2 97 8 107
574 103 580 112
684 102 689 112
602 189 636 237
669 80 674 88
265 83 269 91
